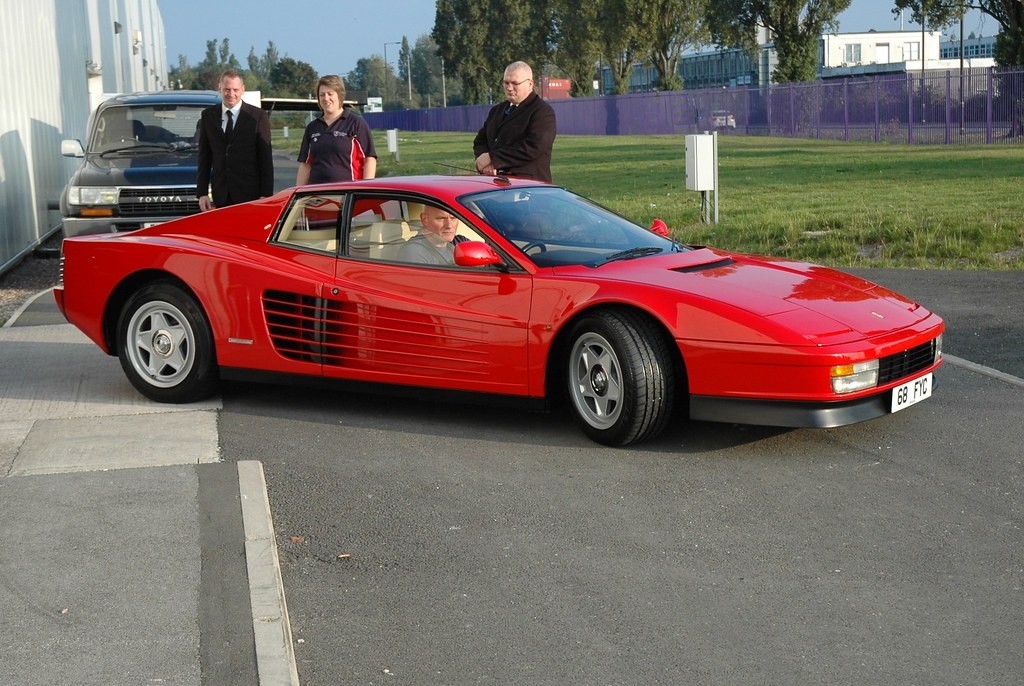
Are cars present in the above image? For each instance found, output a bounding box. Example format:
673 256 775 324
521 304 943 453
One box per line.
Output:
59 90 223 239
708 111 737 133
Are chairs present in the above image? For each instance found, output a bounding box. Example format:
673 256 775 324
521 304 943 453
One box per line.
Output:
112 120 146 142
191 119 201 143
369 220 418 260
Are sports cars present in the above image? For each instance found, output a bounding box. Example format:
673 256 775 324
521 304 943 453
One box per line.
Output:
53 174 944 447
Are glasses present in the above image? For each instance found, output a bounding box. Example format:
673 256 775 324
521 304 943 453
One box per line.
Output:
504 79 530 87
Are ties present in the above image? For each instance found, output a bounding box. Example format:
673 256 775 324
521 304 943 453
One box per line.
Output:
505 105 516 117
225 110 233 140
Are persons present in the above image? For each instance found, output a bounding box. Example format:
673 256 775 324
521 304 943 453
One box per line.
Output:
295 75 377 186
196 69 275 212
473 61 557 183
398 205 495 269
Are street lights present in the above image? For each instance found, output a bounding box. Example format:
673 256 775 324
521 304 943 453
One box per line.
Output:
384 42 401 102
